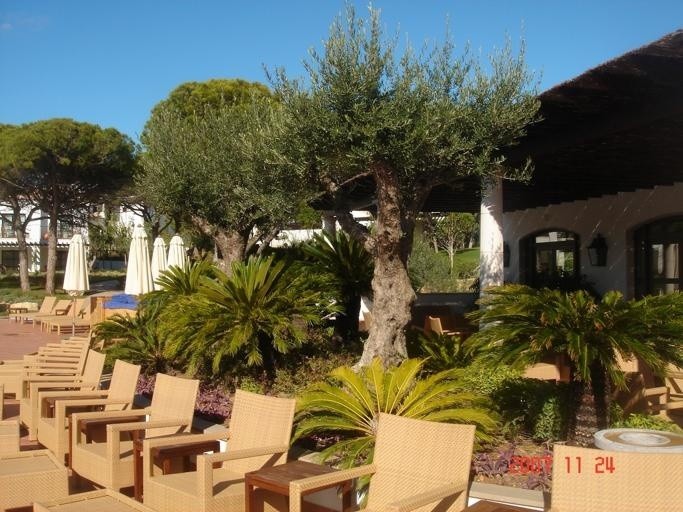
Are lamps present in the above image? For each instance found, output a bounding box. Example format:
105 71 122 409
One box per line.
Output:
586 234 606 267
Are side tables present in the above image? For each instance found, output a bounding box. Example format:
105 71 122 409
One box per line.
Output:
244 459 357 512
462 499 537 512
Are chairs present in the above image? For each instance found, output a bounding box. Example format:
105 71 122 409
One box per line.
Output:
550 444 681 510
8 296 97 337
142 389 296 510
290 412 476 511
0 337 221 511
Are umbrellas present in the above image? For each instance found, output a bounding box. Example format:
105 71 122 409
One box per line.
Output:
150 234 168 291
61 229 90 301
166 231 187 283
123 223 154 298
